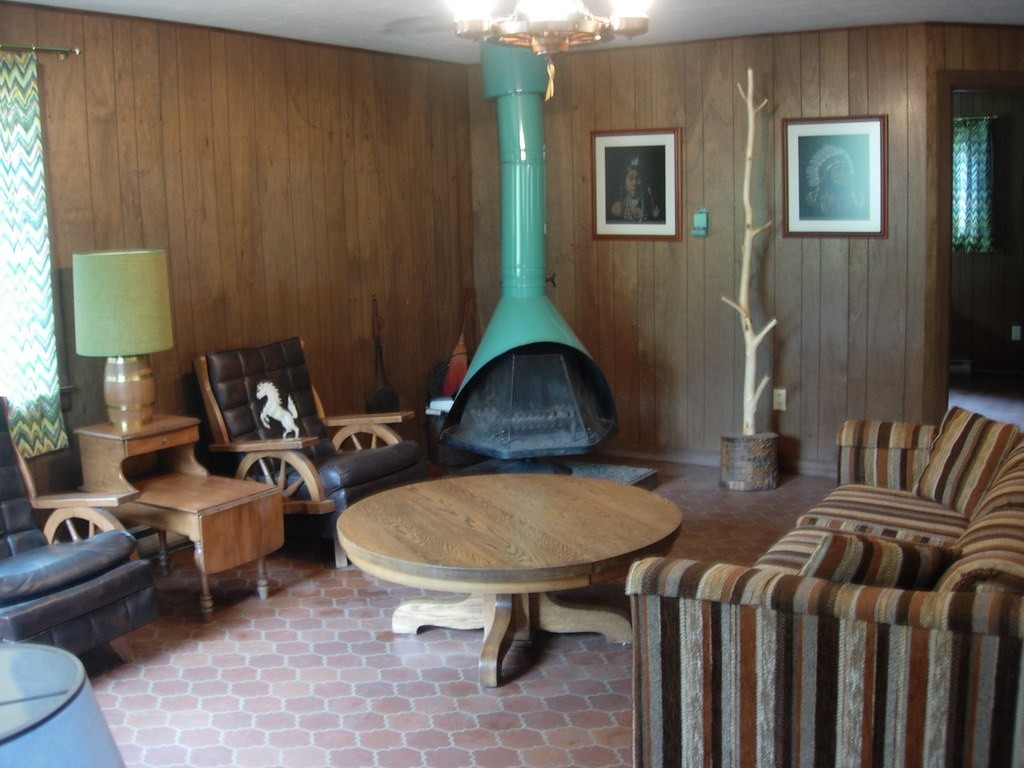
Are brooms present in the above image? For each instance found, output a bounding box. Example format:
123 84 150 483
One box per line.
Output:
442 288 471 396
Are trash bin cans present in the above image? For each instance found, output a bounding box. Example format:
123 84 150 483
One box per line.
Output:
426 398 466 467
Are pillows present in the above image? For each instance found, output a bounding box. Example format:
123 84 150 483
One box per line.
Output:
913 407 1019 524
794 529 963 591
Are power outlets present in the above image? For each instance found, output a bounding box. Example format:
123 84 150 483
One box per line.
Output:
773 388 786 412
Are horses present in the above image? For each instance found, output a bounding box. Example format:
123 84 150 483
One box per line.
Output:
255 379 300 440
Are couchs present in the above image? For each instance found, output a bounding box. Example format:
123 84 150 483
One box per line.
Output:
625 420 1024 768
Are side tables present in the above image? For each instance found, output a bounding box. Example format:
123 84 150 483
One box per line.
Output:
71 412 289 624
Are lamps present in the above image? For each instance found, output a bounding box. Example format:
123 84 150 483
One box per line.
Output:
72 248 175 429
1 640 126 767
443 0 651 103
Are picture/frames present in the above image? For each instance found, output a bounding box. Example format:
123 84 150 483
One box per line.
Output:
781 112 889 238
591 127 683 240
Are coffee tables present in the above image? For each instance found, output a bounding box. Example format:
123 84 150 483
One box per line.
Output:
335 473 684 687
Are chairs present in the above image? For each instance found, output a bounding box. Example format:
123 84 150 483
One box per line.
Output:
190 336 417 568
0 397 161 654
